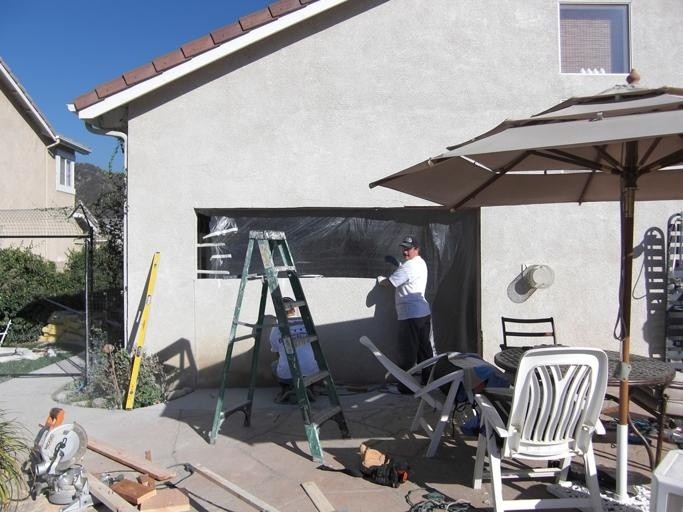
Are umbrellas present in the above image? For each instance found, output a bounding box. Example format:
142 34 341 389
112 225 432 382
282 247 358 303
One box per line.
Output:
368 67 683 511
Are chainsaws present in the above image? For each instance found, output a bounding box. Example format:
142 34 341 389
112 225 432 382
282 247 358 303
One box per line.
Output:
22 408 89 504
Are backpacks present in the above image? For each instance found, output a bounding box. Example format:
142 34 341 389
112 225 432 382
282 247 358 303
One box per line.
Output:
436 356 510 403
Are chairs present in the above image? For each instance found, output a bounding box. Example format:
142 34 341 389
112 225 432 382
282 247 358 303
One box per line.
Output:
472 348 608 512
359 336 485 457
501 317 556 349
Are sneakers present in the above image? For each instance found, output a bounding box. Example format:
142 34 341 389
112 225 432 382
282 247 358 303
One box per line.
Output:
389 385 400 394
306 387 318 401
279 389 292 404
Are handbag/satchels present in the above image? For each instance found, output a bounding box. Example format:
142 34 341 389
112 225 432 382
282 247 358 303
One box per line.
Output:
361 455 411 488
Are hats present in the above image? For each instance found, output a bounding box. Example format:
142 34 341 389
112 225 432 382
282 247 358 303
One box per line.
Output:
399 235 419 248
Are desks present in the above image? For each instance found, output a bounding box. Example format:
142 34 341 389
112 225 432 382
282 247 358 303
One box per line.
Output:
651 450 683 512
495 348 675 476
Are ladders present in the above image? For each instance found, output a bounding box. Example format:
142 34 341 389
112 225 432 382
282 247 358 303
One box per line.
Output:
211 231 351 462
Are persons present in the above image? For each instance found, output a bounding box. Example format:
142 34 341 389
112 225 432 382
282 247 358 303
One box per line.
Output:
268 297 320 405
377 235 434 395
